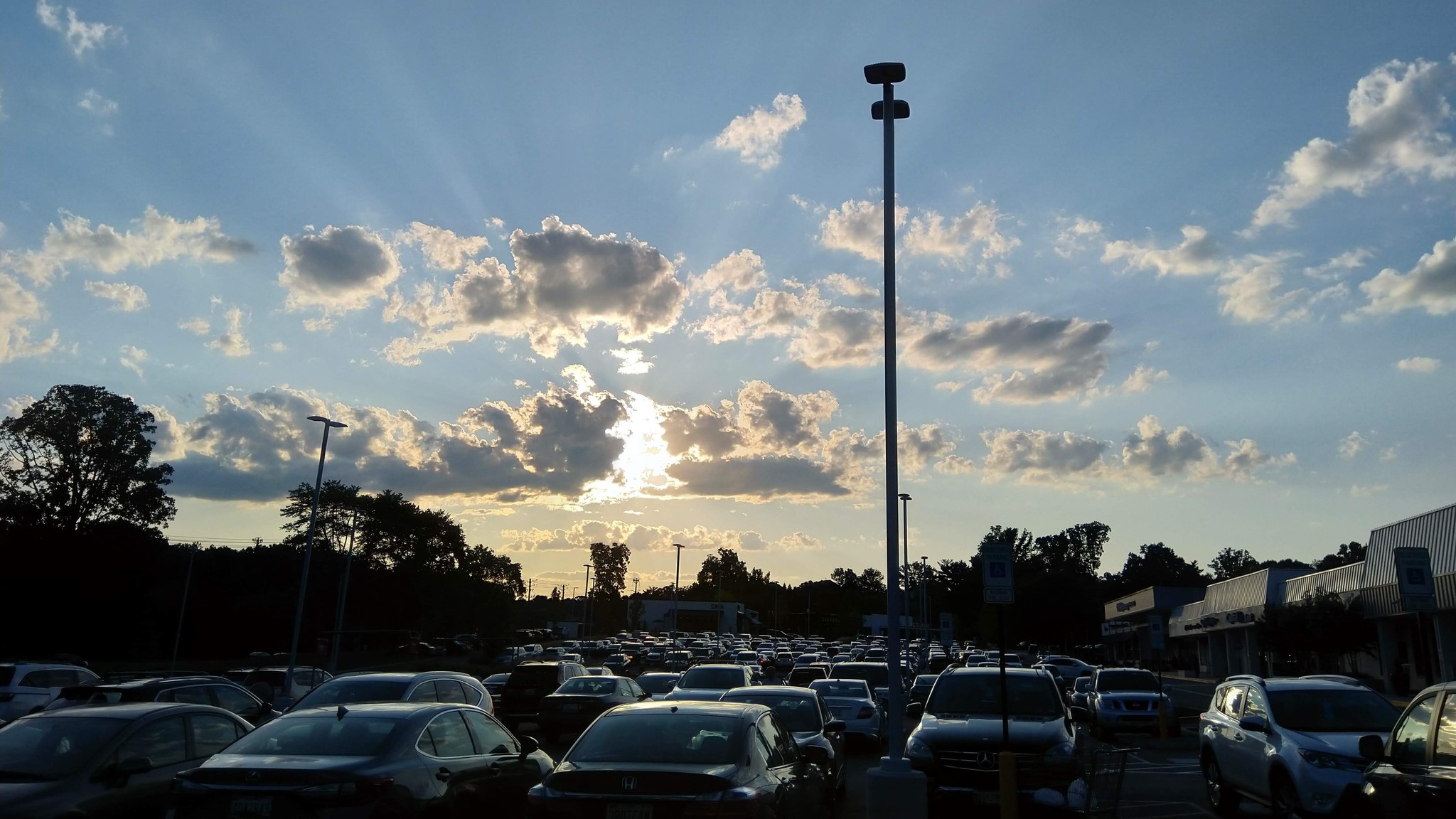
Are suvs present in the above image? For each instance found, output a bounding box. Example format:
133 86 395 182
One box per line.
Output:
1196 674 1407 819
903 661 1090 798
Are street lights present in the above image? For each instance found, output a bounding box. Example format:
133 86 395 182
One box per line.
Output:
921 556 928 651
579 565 595 664
862 59 913 776
774 581 779 649
716 570 724 664
898 493 913 690
672 543 686 673
590 578 597 641
571 587 579 622
281 415 349 699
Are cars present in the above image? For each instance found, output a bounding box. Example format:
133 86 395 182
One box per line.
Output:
161 699 559 819
522 698 832 819
0 699 266 819
1 626 1176 819
1355 680 1456 819
